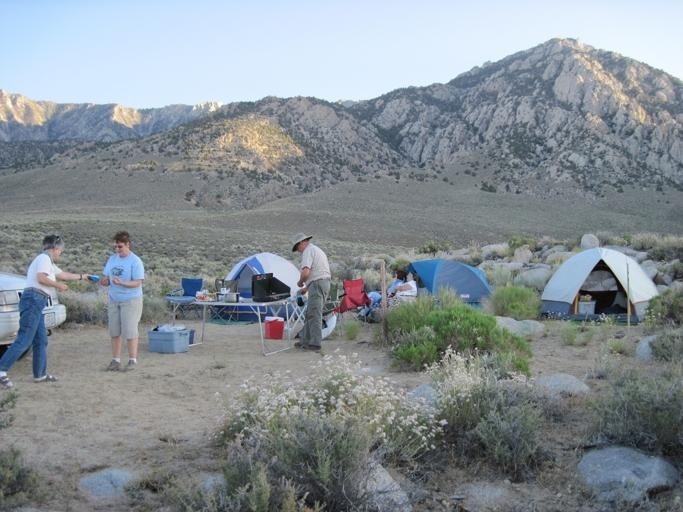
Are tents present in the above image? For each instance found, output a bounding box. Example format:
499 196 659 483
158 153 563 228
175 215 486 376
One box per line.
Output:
210 252 308 323
534 246 660 326
406 259 493 311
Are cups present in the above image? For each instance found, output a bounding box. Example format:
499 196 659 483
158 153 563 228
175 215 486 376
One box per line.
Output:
221 288 226 294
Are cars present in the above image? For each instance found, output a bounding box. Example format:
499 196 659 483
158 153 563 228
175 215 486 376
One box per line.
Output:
0 273 67 362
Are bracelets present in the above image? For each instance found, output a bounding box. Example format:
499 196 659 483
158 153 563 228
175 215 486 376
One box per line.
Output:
79 274 82 281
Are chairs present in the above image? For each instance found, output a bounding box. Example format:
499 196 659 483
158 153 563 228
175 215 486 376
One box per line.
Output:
290 299 309 332
167 278 203 318
333 278 371 325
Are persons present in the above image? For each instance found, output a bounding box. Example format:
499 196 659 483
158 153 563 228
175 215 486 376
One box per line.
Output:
100 230 145 373
389 272 419 299
291 232 331 351
0 234 94 390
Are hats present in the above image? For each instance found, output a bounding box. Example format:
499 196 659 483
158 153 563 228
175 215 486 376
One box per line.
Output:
292 233 312 251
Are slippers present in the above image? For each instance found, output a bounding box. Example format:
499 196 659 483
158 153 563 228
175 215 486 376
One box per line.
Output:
0 376 13 387
34 374 58 382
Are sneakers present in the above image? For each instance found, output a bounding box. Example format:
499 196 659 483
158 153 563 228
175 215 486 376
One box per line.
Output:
107 358 137 371
295 342 321 352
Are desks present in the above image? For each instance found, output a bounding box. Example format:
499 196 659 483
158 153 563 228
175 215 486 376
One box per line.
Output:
192 297 293 357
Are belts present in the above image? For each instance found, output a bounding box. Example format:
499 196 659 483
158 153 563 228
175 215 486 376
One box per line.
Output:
24 288 49 298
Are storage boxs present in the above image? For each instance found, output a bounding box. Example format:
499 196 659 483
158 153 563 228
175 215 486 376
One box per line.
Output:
578 300 595 316
188 329 196 346
149 329 191 354
